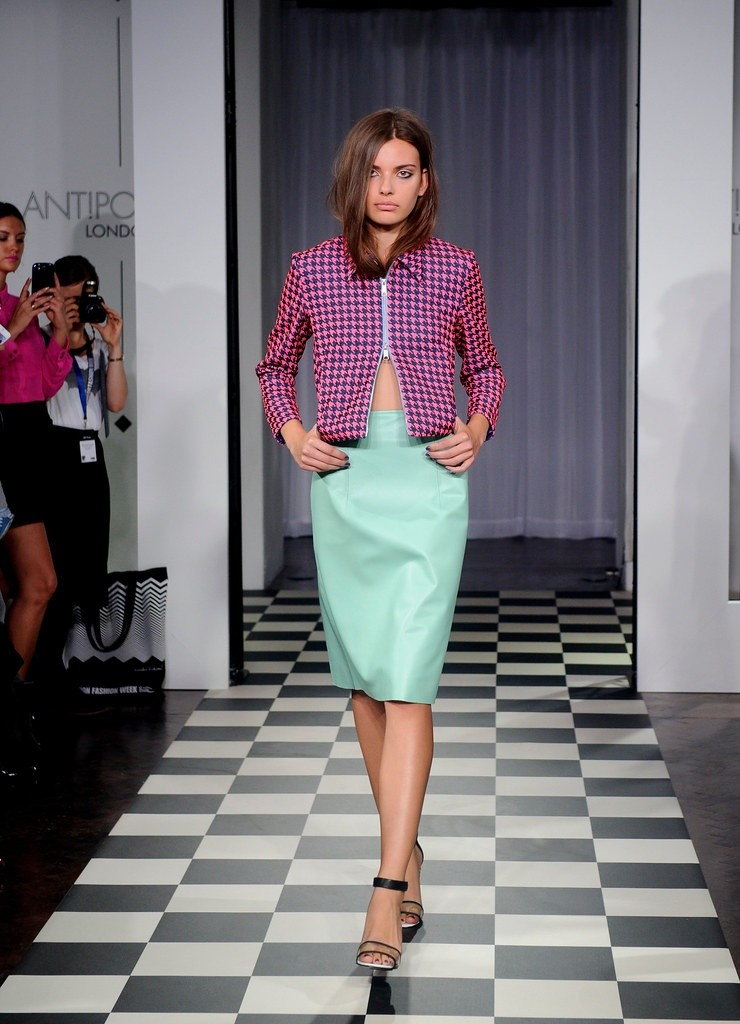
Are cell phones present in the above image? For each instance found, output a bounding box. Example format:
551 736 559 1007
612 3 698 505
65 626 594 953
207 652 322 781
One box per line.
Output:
32 263 55 308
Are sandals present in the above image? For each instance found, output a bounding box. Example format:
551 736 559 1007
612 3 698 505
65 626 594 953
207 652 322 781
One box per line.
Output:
402 834 424 933
356 877 408 970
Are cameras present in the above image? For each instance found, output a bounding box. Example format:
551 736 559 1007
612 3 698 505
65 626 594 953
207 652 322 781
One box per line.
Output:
73 279 106 323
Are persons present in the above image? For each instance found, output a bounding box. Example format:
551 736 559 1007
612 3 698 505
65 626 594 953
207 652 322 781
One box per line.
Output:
0 202 127 678
255 109 506 968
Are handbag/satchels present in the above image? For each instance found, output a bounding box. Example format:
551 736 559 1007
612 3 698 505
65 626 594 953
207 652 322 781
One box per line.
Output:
61 567 168 696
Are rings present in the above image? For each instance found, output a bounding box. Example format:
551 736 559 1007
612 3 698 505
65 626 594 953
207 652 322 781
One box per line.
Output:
31 303 36 310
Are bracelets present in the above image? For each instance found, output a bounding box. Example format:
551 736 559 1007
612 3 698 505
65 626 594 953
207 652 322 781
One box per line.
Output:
107 355 124 362
